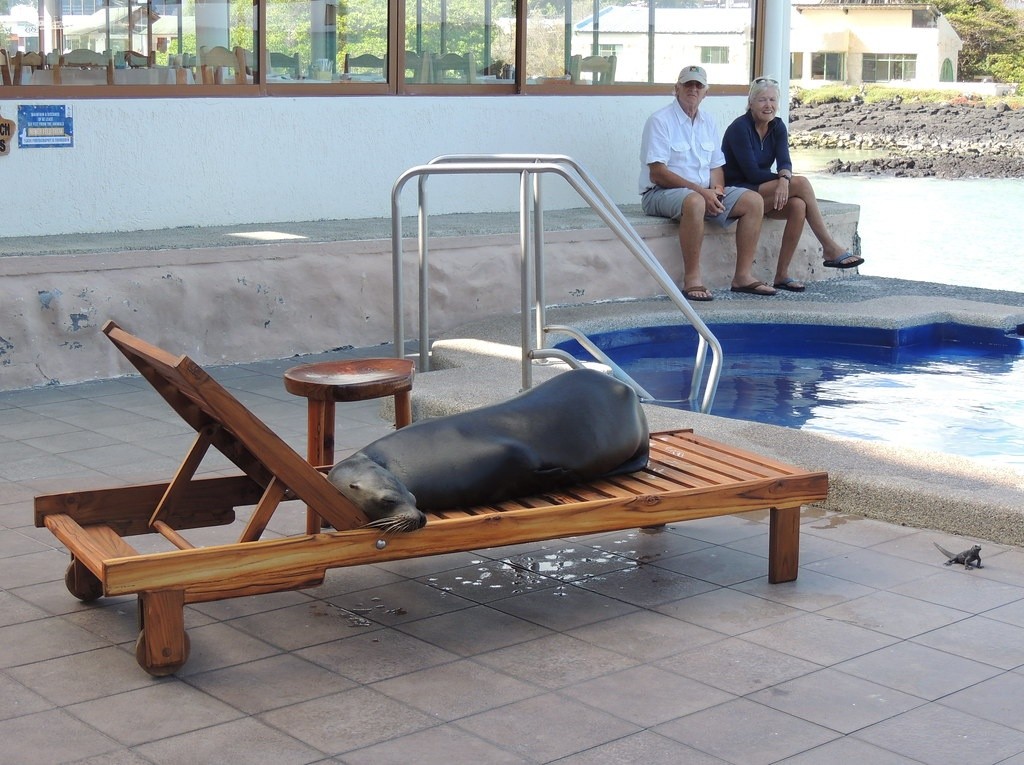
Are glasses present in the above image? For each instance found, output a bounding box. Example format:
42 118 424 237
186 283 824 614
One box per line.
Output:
681 82 704 89
750 79 778 94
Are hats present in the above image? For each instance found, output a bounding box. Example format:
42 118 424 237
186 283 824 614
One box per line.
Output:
678 66 707 85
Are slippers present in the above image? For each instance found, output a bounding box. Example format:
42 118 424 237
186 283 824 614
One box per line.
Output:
823 252 864 268
774 277 805 292
730 281 777 296
682 286 714 301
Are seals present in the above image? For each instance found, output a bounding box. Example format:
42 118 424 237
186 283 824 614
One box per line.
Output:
324 369 650 533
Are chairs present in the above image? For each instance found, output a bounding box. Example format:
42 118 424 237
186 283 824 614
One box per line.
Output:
0 45 617 84
34 318 829 680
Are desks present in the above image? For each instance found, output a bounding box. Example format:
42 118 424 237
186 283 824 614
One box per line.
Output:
478 75 593 84
30 68 196 84
282 357 415 534
224 74 386 83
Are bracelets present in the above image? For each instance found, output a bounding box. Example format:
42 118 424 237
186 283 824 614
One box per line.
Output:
715 185 723 191
779 175 790 183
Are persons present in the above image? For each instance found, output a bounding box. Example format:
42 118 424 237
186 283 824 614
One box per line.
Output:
638 65 777 301
721 77 865 291
